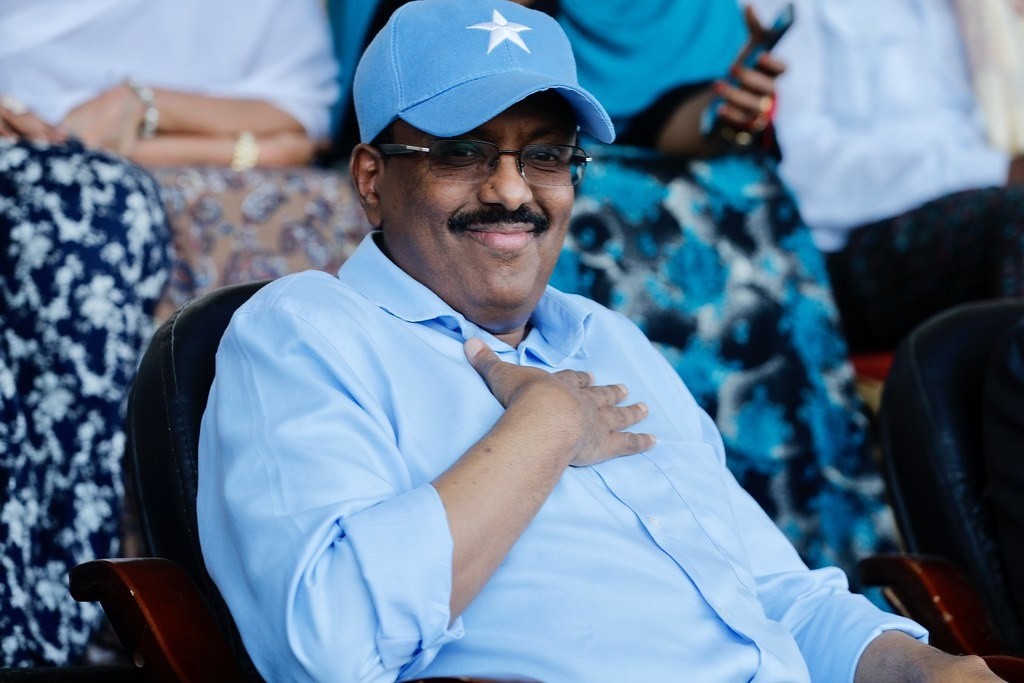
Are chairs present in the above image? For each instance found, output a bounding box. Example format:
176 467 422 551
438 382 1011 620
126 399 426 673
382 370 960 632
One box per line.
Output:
68 281 527 683
857 296 1024 683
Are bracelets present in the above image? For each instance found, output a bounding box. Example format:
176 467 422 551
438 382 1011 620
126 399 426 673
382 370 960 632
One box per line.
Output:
232 132 259 169
135 84 158 138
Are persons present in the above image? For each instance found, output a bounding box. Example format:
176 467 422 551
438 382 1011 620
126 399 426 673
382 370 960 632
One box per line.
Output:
0 0 1024 683
195 0 1004 682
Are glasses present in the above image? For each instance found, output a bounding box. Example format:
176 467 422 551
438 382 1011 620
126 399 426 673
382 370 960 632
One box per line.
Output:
377 138 593 187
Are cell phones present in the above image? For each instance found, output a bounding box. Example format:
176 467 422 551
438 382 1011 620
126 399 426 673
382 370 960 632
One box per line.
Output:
700 4 794 134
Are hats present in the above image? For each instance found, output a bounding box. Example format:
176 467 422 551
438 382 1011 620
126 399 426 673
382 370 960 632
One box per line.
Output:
353 0 617 146
